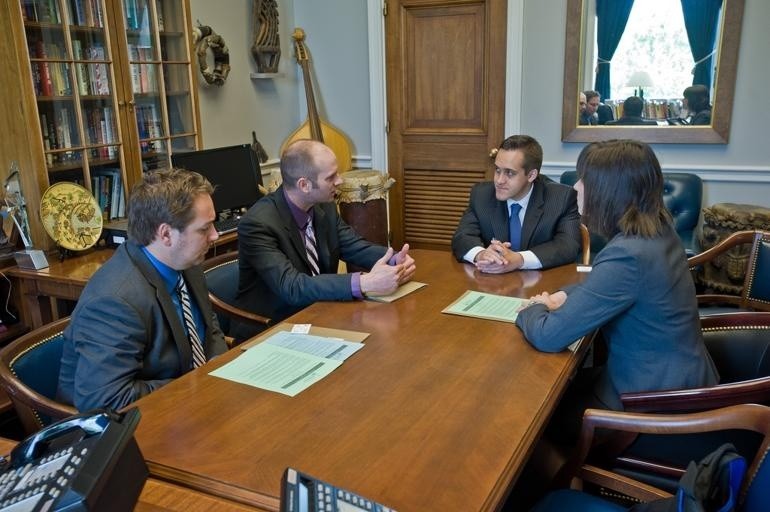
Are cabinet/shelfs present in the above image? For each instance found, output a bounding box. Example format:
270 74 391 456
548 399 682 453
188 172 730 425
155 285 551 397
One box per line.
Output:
0 0 203 259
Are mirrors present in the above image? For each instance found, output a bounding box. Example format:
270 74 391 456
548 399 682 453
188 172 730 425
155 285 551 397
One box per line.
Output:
561 0 744 144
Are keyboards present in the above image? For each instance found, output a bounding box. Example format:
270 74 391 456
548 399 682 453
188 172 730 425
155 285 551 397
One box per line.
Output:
213 215 247 235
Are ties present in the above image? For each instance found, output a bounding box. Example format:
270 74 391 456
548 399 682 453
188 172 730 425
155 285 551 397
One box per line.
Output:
508 203 521 252
304 215 320 276
175 273 207 371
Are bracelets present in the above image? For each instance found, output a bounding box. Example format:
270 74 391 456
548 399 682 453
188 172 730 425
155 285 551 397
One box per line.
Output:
526 299 543 307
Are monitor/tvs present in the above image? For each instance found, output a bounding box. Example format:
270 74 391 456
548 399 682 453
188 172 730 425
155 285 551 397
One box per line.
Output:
171 143 266 217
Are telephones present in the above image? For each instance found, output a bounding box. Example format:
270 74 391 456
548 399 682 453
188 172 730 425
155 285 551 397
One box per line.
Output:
0 406 150 512
279 467 399 512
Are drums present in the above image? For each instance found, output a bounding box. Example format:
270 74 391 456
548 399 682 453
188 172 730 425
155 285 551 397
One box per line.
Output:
337 168 390 273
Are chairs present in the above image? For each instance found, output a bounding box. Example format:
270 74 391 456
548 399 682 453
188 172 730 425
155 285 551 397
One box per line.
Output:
500 402 770 512
0 316 237 436
200 248 272 345
586 310 770 475
559 170 704 285
597 230 770 488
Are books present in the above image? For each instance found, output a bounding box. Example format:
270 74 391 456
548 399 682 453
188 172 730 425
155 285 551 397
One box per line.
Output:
23 0 166 222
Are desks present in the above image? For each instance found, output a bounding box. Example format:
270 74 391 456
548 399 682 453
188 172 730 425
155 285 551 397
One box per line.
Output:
114 247 605 512
4 225 239 333
0 437 265 511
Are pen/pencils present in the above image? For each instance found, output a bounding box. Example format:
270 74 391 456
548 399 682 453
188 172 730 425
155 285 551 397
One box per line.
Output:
492 237 502 258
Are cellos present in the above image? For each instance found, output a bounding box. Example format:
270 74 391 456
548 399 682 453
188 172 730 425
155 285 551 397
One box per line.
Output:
281 29 351 175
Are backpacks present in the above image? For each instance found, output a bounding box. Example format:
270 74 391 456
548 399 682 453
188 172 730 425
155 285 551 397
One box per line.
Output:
629 443 747 512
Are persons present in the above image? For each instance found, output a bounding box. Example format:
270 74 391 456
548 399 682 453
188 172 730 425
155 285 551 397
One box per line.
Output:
579 90 601 125
579 92 587 113
683 84 712 126
513 137 722 479
50 167 230 426
228 139 417 347
451 134 581 276
605 95 658 126
